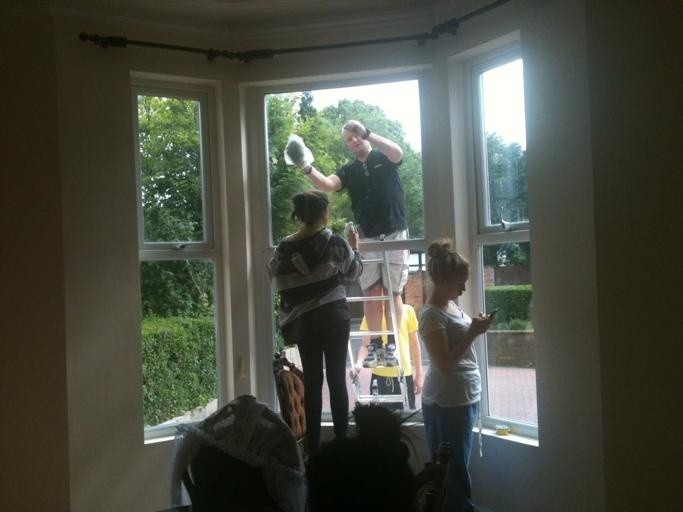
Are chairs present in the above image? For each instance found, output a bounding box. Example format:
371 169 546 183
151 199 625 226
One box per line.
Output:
270 351 308 464
167 391 310 512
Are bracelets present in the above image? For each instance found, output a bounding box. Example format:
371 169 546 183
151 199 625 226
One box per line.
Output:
304 165 312 174
352 248 359 250
362 128 371 139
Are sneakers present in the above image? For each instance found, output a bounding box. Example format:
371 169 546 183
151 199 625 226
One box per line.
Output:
383 343 399 367
362 343 385 369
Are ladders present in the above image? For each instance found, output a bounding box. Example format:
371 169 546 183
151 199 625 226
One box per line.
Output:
346 234 409 412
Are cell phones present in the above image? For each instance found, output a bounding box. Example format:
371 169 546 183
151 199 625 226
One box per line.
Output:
488 310 498 320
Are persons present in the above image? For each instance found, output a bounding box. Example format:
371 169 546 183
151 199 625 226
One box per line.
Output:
305 402 416 512
349 286 422 410
268 190 363 466
418 239 495 500
287 120 409 368
